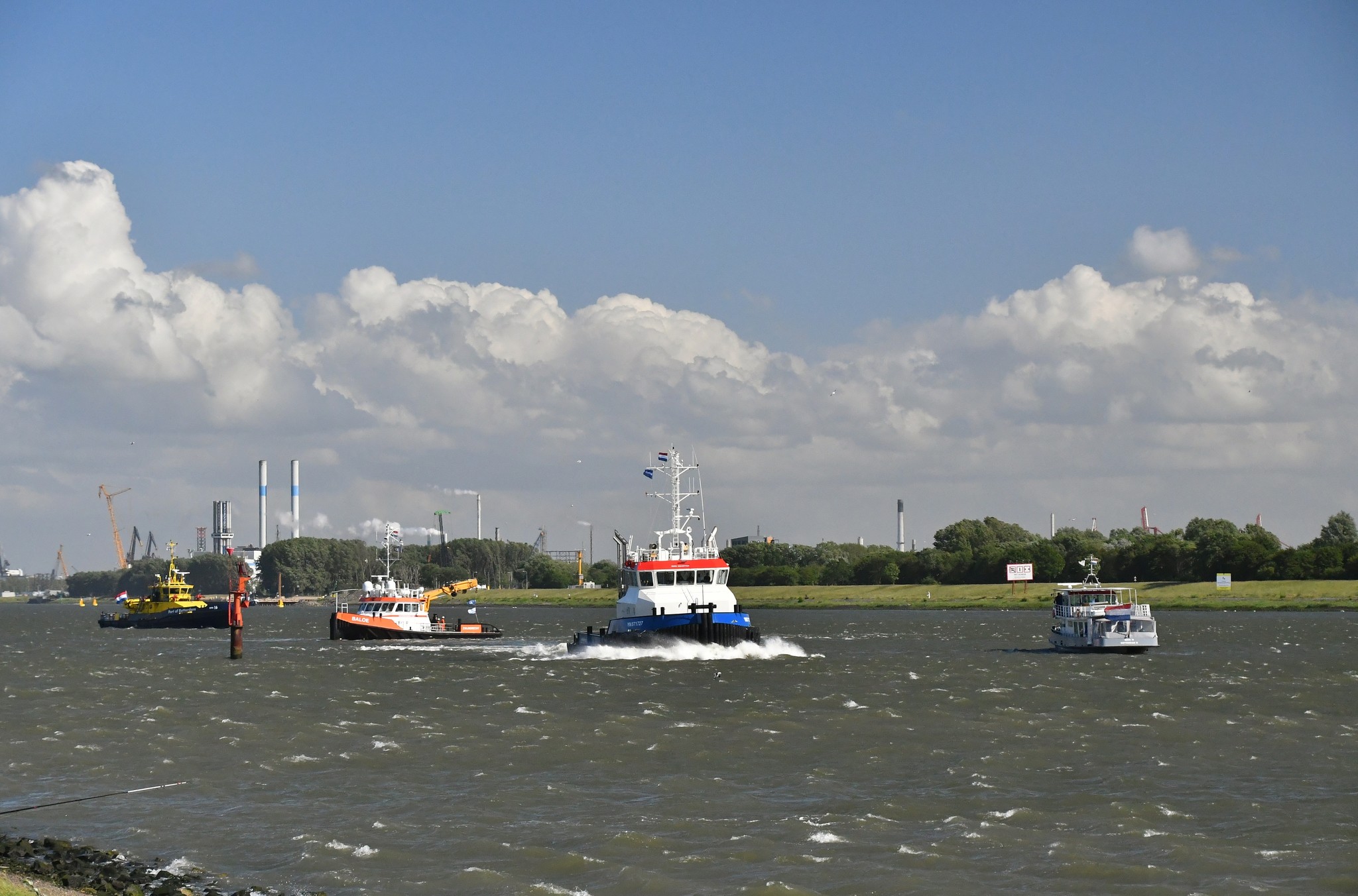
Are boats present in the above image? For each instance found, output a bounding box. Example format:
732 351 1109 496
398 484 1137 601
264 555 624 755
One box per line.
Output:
98 540 234 629
27 596 51 604
567 444 761 652
328 523 503 640
1049 553 1160 654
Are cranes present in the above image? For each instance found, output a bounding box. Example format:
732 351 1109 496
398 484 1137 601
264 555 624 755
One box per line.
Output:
53 545 68 578
1140 506 1158 536
434 510 455 569
98 484 158 569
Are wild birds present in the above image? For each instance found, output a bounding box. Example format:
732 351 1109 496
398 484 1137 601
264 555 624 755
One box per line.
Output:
831 389 837 397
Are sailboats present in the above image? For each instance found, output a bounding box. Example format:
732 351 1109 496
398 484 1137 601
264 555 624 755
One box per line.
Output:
277 599 284 608
92 597 98 606
79 596 85 607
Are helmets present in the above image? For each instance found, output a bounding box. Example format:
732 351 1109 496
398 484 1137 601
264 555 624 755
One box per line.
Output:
437 618 440 620
442 616 445 618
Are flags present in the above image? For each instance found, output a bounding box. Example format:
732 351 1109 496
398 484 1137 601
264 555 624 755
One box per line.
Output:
468 607 477 614
658 452 668 462
1104 603 1131 621
467 599 476 605
643 469 653 480
114 592 128 604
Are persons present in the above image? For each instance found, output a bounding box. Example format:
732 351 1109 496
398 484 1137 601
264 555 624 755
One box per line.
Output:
437 618 441 631
441 616 445 631
1056 592 1064 613
